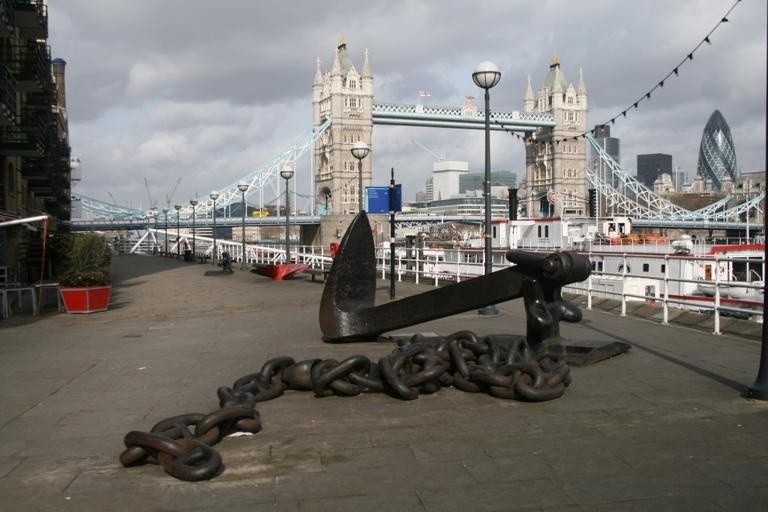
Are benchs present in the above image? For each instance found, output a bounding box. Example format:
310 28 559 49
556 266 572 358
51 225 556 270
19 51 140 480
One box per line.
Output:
159 252 167 256
197 256 211 264
302 268 330 283
168 253 176 257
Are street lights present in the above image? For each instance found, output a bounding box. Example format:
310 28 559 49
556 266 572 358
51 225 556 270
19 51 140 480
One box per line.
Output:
189 197 198 262
279 165 295 264
471 59 503 315
153 210 159 255
237 180 250 270
209 190 219 265
162 207 170 258
174 202 182 260
350 140 371 214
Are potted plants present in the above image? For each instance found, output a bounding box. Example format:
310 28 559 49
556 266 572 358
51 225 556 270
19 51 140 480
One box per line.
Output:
59 270 112 314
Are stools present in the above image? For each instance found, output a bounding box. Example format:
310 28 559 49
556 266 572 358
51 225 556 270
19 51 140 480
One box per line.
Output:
34 280 64 313
0 264 36 318
